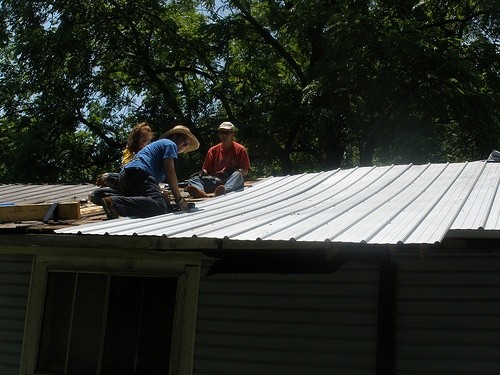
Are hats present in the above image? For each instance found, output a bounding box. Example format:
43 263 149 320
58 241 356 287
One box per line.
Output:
159 125 200 153
217 122 234 129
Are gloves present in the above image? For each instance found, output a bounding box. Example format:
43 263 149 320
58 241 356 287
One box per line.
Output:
177 197 189 210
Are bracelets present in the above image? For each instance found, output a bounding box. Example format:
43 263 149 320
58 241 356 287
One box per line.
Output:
175 197 185 206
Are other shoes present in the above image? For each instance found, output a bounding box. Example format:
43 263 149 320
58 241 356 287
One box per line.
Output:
215 185 226 197
101 197 119 219
187 186 209 198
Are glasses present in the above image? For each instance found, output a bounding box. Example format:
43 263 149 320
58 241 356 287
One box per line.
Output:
219 130 234 135
184 138 190 147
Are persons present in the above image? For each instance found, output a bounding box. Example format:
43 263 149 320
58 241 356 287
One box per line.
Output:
102 126 200 219
89 122 153 205
188 122 250 198
